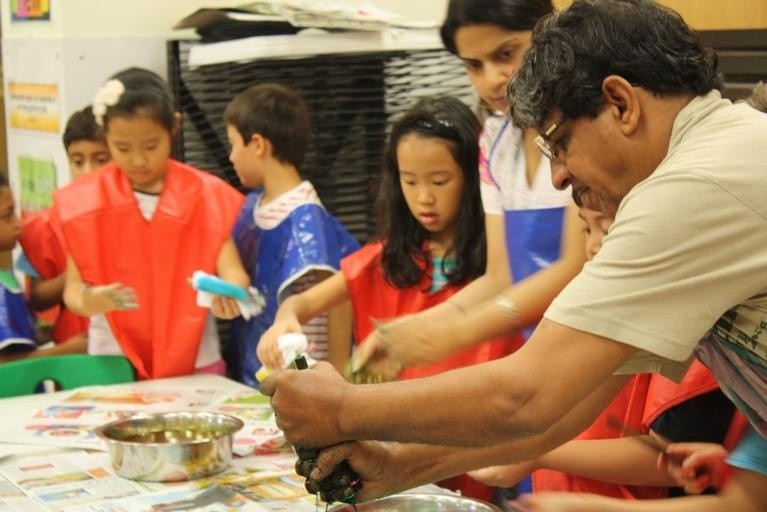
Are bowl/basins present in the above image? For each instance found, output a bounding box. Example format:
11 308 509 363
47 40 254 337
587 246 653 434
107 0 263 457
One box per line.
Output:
94 410 244 483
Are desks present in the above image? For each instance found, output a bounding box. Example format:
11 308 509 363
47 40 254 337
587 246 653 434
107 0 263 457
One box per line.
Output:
0 374 460 510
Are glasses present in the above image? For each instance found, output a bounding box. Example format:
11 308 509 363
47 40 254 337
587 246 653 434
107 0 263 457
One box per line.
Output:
534 115 571 161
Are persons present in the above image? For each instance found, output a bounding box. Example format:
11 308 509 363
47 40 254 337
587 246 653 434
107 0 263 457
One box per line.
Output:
216 80 372 408
13 101 114 344
255 0 767 512
1 171 89 364
50 66 252 384
